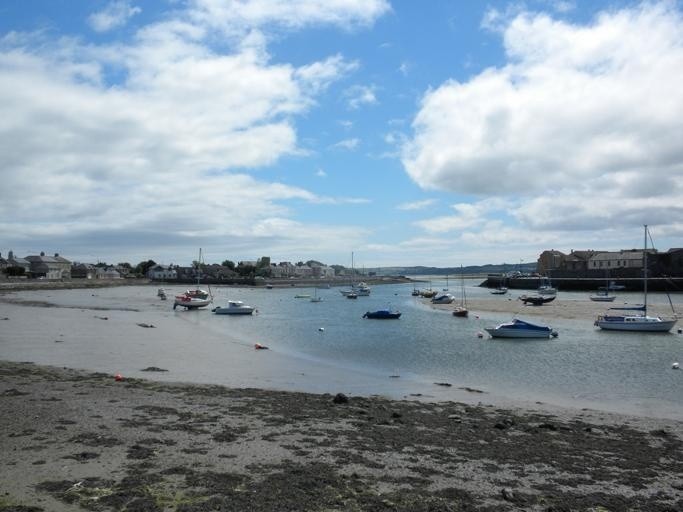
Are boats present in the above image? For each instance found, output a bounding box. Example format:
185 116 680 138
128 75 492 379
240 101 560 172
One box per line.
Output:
431 294 454 304
210 300 254 315
489 284 507 295
598 281 626 291
362 308 401 319
517 295 556 303
482 318 558 339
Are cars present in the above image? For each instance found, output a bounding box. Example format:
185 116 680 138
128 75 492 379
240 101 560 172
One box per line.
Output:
507 270 521 277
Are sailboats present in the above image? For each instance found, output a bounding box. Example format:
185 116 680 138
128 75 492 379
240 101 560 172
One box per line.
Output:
452 263 469 316
266 251 448 303
174 247 211 308
593 222 678 332
589 269 615 301
536 258 558 295
